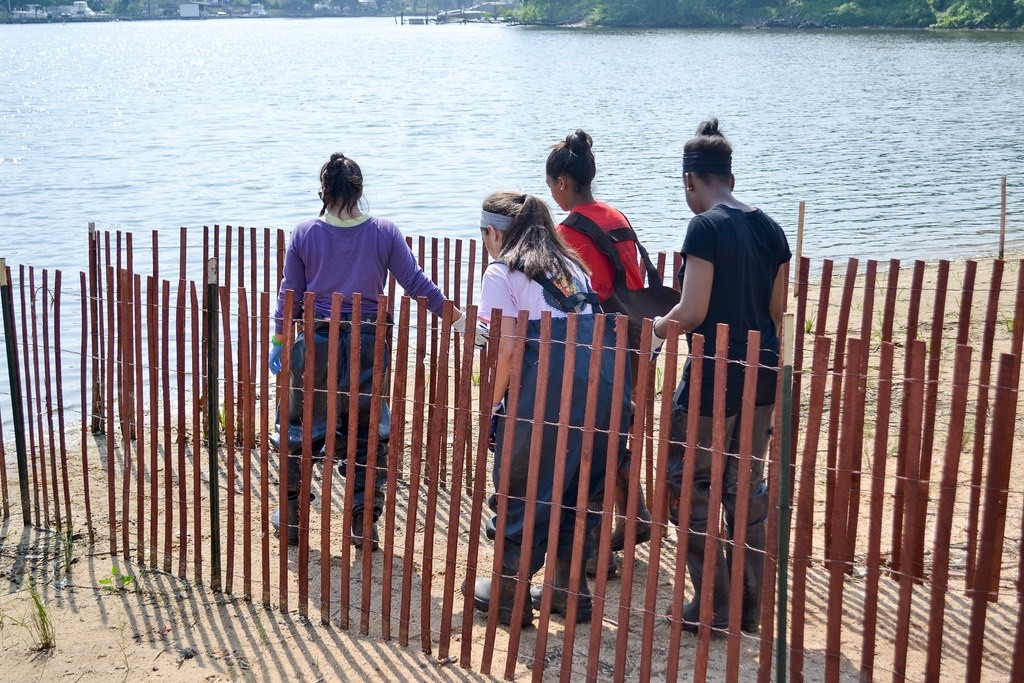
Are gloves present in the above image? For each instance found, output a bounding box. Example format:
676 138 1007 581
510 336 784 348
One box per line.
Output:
486 403 506 452
450 312 490 350
267 337 283 374
650 316 665 361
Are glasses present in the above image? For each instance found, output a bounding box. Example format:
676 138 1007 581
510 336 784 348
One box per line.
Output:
317 185 322 201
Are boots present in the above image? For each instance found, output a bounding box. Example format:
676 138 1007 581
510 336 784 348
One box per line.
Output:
724 533 768 632
582 514 617 580
668 516 731 628
530 555 593 622
349 513 379 550
608 452 652 551
460 559 534 627
271 499 300 545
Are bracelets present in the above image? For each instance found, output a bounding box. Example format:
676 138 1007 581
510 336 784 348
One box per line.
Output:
272 336 282 346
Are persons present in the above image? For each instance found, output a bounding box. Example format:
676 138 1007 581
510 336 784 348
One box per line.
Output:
268 153 491 551
649 117 792 635
546 129 681 580
461 192 632 627
